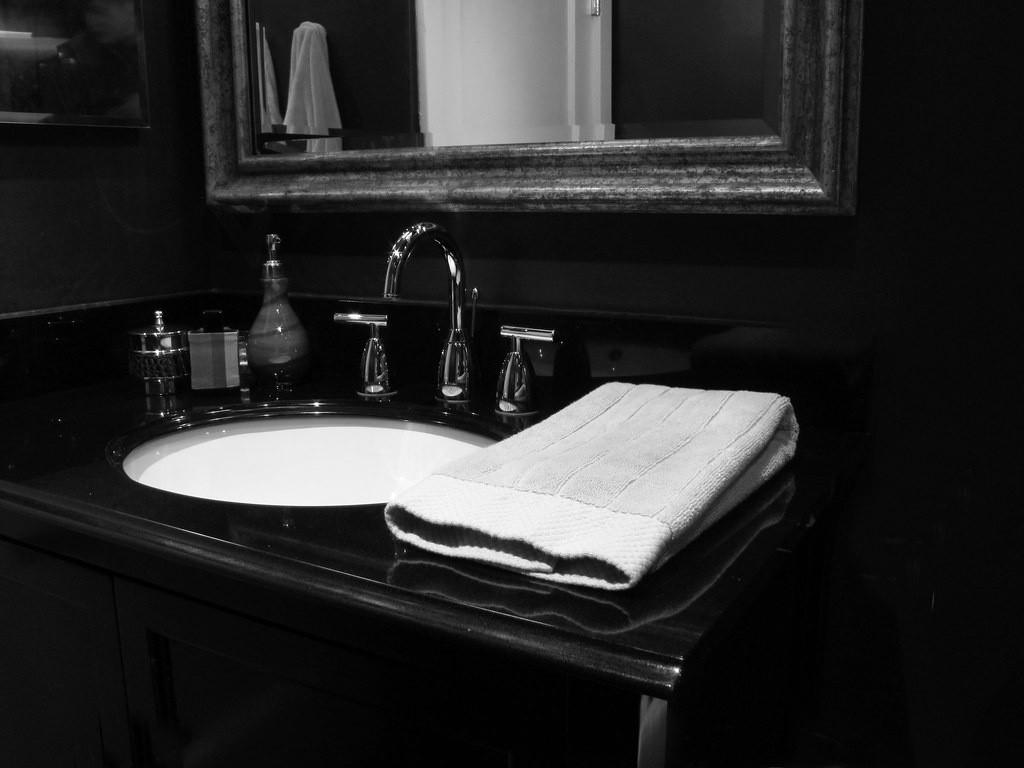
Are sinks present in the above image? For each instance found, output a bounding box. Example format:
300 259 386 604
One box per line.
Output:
120 407 508 509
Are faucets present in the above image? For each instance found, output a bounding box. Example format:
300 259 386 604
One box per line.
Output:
382 219 480 404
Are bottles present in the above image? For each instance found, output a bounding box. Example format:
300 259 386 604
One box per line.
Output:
246 233 304 401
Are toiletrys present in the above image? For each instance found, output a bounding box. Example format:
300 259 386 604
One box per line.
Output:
246 232 312 375
188 310 242 392
128 308 190 391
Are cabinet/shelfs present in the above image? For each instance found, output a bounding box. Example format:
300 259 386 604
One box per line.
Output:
0 504 666 768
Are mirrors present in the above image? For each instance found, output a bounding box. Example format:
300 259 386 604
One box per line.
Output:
194 0 860 216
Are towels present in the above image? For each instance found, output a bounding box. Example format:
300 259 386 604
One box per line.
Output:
390 476 797 638
384 380 800 591
280 20 345 153
